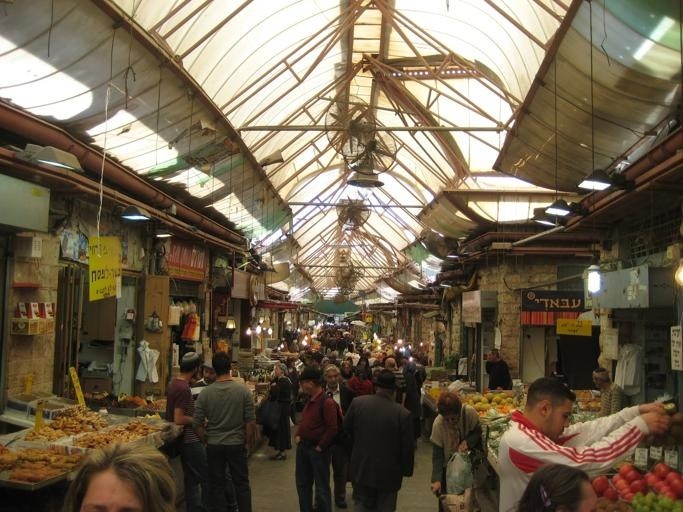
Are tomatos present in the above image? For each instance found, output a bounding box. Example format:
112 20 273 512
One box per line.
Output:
592 463 683 502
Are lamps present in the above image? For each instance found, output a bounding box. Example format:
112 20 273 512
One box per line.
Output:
154 227 176 239
584 0 602 297
581 168 627 194
530 207 556 228
545 197 584 221
219 317 236 339
16 141 84 177
115 203 152 225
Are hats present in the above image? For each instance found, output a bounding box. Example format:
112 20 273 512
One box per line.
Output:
201 359 214 371
182 352 199 362
374 370 395 387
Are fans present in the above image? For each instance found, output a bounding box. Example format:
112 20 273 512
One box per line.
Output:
321 92 398 308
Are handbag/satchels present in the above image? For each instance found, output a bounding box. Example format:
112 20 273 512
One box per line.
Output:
469 448 497 489
439 487 480 512
259 399 280 418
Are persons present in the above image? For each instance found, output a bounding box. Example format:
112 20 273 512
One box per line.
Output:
591 366 631 417
163 321 516 511
60 438 181 512
496 373 681 512
517 460 601 512
